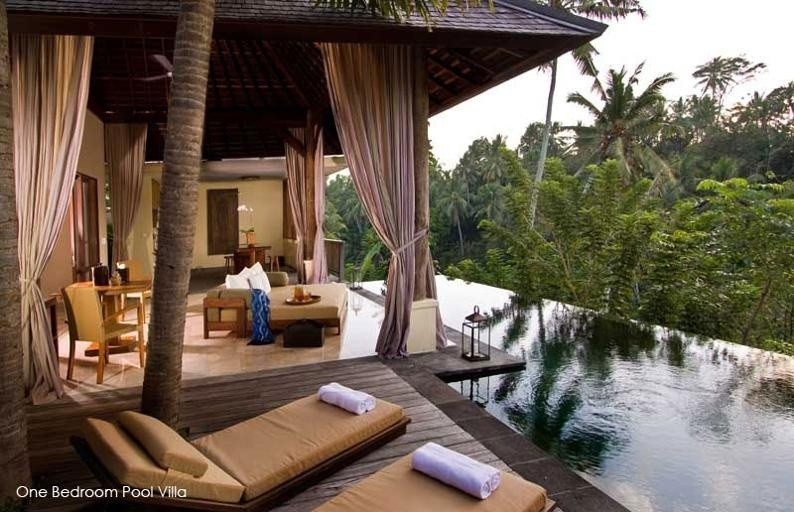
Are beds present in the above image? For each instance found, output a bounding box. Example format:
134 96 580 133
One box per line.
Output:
202 271 350 340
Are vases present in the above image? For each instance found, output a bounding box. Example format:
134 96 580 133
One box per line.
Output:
245 232 256 246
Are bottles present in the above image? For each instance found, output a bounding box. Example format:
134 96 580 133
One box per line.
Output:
94 266 109 286
110 273 122 286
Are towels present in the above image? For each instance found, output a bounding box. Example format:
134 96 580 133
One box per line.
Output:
315 381 376 415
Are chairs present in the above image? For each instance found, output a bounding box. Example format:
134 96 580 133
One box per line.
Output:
60 259 152 386
67 394 566 512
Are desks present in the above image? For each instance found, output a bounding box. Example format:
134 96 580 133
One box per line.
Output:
50 275 152 355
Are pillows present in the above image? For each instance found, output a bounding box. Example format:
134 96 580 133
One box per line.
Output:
225 260 272 296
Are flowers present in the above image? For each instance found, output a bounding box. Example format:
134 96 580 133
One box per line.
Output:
236 204 256 235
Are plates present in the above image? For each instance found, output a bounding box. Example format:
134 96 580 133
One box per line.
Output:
284 295 321 306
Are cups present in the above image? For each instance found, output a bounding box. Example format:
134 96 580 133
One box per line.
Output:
294 285 304 302
